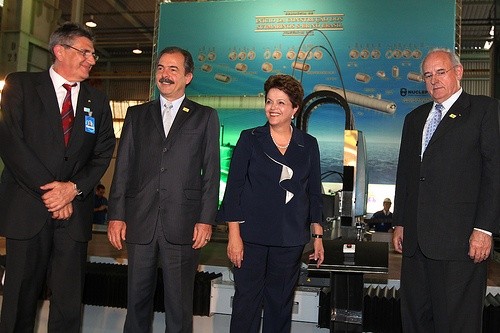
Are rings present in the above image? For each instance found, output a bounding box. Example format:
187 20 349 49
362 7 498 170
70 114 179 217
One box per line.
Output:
205 239 210 241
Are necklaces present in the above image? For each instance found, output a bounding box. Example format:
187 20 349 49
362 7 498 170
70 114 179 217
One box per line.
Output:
271 125 293 148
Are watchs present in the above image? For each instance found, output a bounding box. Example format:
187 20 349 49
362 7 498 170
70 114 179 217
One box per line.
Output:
69 181 83 196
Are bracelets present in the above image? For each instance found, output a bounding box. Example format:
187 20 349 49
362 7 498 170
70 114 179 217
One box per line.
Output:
311 234 323 238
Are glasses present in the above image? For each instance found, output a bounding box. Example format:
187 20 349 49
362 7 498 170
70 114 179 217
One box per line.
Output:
423 65 458 83
63 44 99 62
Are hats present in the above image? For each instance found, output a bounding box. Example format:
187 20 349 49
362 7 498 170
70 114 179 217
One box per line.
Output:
384 198 391 202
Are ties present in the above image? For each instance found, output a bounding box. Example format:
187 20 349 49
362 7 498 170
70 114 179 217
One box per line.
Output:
163 102 174 136
60 83 75 146
424 103 445 150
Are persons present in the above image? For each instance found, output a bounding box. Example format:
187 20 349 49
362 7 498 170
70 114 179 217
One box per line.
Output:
216 75 327 333
0 20 118 332
105 46 220 333
392 47 500 333
368 197 394 232
91 184 108 225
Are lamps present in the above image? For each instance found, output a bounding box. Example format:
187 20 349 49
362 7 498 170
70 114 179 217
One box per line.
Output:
85 14 97 28
132 42 143 55
